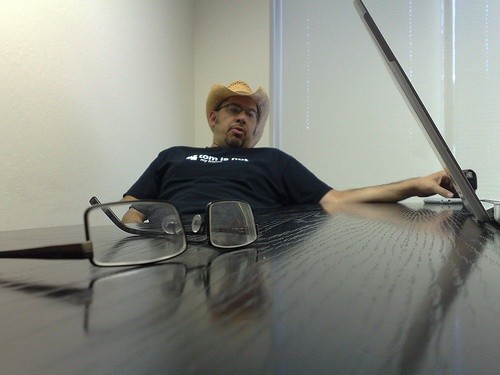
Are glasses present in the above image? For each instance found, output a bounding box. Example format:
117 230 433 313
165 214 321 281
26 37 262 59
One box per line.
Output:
0 197 259 268
0 247 259 335
215 104 257 119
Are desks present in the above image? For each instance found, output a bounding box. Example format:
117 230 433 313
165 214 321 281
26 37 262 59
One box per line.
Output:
0 198 500 375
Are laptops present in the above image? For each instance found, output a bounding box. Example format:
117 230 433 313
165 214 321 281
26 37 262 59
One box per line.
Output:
353 0 500 223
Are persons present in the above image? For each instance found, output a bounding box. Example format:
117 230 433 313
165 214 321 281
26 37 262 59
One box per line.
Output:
121 80 453 226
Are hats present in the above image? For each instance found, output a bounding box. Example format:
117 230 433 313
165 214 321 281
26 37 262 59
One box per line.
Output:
205 80 270 148
